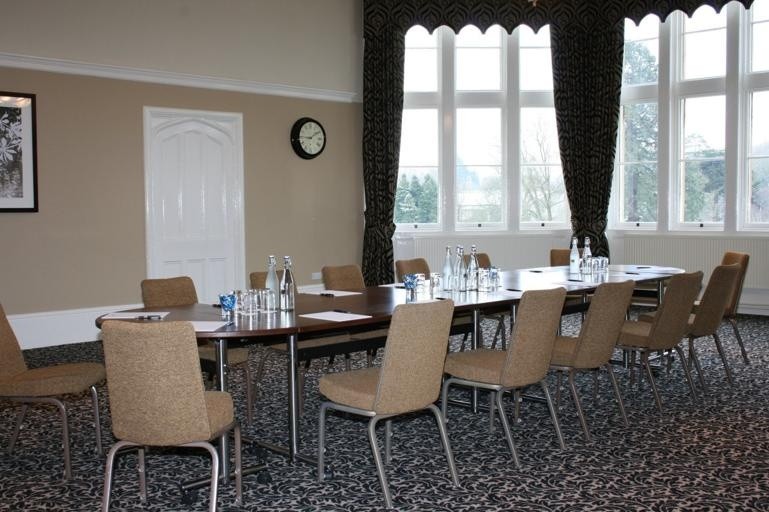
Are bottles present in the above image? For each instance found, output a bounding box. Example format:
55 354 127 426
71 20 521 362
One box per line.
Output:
568 236 582 272
582 237 594 260
265 255 282 308
280 256 296 311
443 245 480 290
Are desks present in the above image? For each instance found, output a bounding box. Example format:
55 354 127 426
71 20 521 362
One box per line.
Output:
97 265 686 472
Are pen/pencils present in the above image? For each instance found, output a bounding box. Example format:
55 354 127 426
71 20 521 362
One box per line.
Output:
333 309 351 313
436 298 446 300
530 271 542 272
568 279 583 282
626 273 639 274
321 293 334 297
637 267 651 268
395 286 405 288
507 289 522 291
134 315 161 320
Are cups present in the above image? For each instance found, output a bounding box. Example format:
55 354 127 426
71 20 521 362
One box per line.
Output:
221 289 277 314
581 256 609 277
222 311 294 333
402 268 502 294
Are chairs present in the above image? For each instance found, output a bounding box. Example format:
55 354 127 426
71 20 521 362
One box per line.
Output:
619 271 699 413
248 269 349 407
1 307 105 481
323 251 502 404
442 287 565 469
317 297 460 509
523 279 629 439
140 276 251 434
103 320 242 512
638 250 749 396
550 250 586 335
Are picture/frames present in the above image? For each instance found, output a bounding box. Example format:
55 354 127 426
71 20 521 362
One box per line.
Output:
0 91 38 212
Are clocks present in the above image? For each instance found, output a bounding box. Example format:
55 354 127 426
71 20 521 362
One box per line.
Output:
290 117 326 160
